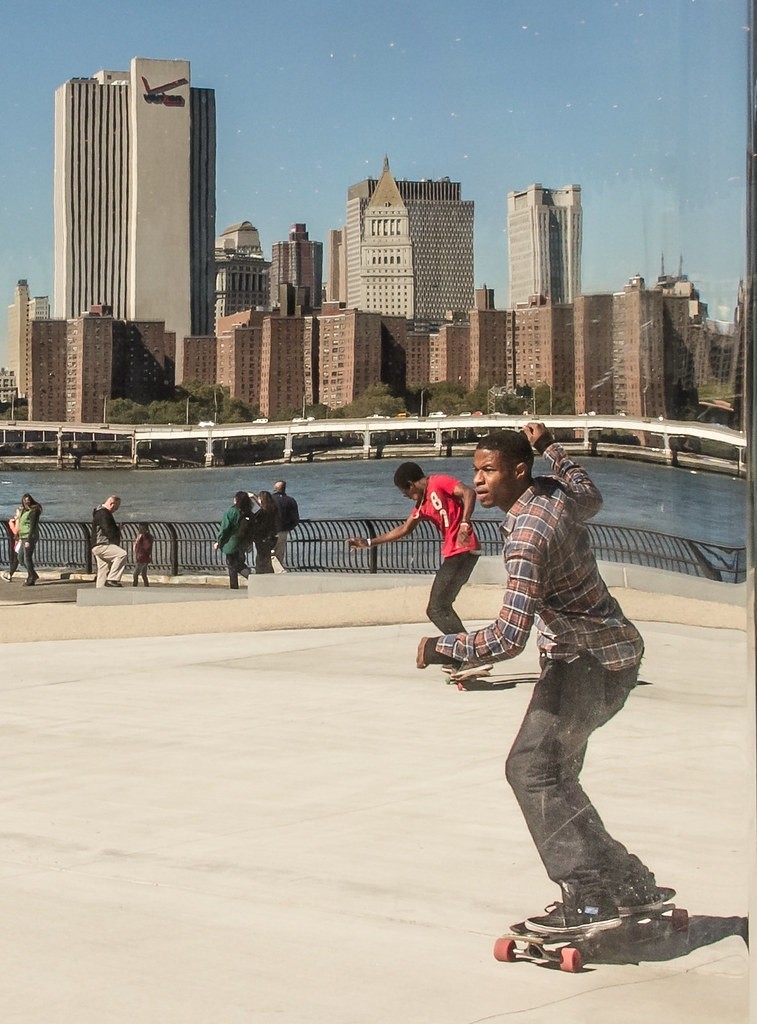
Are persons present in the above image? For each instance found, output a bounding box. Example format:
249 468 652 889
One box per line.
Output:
0 494 41 586
417 423 661 934
132 523 154 587
91 495 127 589
214 481 299 589
345 462 482 673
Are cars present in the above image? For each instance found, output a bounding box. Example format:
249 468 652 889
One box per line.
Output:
366 413 391 420
459 411 472 417
252 418 268 424
617 411 627 417
198 420 215 429
292 416 316 423
473 411 483 416
578 411 596 417
428 412 447 418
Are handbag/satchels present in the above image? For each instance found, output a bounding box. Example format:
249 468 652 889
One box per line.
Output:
15 538 24 553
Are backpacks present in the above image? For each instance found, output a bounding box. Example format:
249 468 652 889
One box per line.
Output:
230 509 255 539
277 495 298 530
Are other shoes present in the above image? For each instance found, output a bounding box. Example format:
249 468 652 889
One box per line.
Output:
1 572 12 583
22 574 39 586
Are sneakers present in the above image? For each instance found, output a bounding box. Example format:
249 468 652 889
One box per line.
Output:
606 882 662 913
441 663 453 673
104 580 123 586
524 902 623 933
450 661 493 678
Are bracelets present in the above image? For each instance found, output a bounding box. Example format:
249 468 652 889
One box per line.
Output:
366 538 372 550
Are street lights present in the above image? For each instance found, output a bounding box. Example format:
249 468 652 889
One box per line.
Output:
532 387 538 420
642 390 650 420
11 395 16 422
212 381 225 423
186 395 193 425
104 395 109 426
420 389 427 418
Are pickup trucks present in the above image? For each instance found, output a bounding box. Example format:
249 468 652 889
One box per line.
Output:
393 413 419 420
489 412 508 417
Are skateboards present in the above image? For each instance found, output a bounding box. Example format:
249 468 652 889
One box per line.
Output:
446 668 491 691
492 885 690 973
